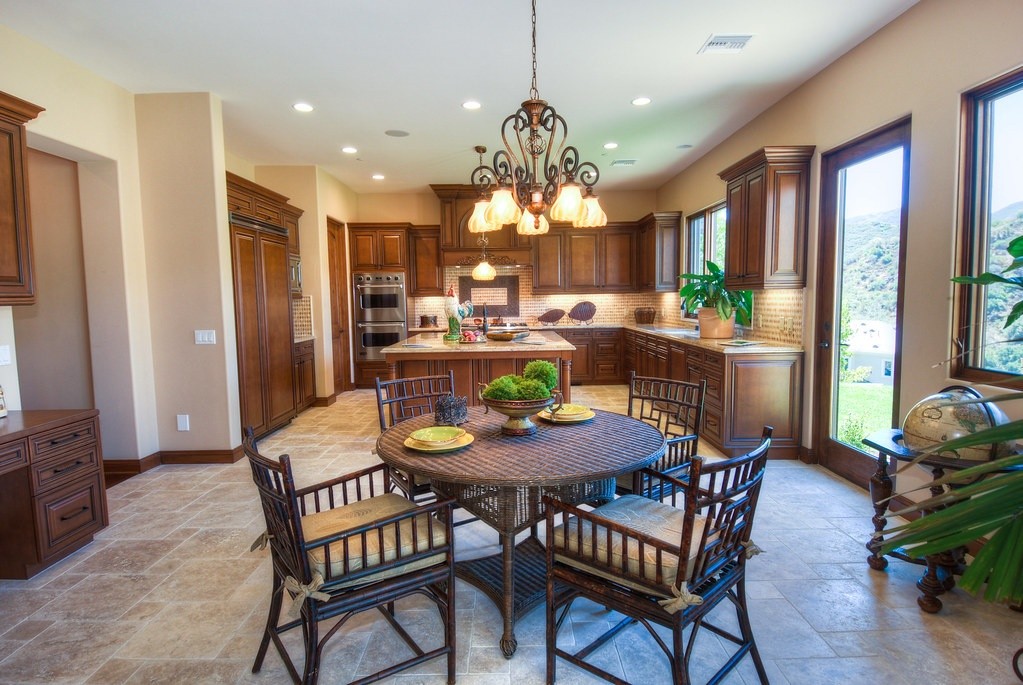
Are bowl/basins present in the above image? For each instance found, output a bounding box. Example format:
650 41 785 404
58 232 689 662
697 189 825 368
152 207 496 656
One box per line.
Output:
543 403 590 419
409 427 466 447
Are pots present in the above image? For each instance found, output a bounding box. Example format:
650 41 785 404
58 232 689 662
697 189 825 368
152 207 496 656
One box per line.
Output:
493 315 503 323
420 316 437 325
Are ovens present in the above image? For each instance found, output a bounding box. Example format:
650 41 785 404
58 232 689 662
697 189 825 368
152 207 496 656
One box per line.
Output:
354 272 407 362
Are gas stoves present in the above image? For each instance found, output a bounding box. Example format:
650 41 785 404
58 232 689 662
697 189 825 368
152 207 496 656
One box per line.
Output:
490 323 527 326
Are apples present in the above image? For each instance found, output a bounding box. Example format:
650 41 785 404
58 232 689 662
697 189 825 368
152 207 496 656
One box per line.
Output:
463 330 480 341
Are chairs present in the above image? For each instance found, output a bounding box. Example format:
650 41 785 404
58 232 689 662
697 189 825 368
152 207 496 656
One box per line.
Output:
542 426 773 685
242 423 457 685
375 369 481 527
615 370 707 515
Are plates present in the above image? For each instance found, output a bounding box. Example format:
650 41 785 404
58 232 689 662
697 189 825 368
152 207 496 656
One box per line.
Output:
537 411 595 423
458 336 487 343
403 433 474 453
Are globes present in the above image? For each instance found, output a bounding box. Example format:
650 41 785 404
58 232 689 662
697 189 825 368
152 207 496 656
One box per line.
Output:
861 384 1023 614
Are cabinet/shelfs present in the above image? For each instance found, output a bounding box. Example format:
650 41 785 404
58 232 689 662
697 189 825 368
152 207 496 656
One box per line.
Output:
0 90 47 307
348 183 804 460
716 145 815 291
226 169 316 441
0 409 110 579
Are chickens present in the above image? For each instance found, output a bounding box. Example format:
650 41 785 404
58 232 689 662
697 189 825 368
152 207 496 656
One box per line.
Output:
445 284 474 335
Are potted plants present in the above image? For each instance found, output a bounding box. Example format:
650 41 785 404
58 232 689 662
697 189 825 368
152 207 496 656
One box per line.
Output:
678 261 751 339
477 359 564 436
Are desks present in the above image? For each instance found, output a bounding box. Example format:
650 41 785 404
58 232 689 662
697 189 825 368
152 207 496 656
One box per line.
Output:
376 405 667 659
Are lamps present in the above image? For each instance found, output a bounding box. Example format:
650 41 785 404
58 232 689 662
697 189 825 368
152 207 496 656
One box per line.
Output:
471 231 497 281
471 0 608 236
468 147 504 233
